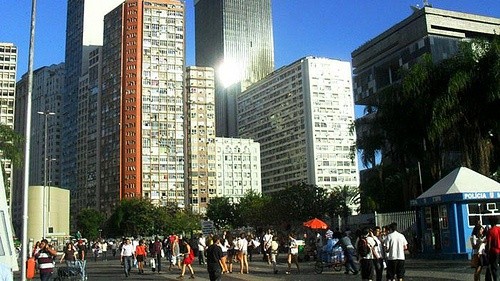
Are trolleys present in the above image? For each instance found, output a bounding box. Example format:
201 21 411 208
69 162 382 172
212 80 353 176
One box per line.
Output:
314 244 345 273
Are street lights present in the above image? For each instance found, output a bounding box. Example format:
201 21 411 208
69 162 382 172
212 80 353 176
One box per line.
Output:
36 110 55 239
42 155 57 238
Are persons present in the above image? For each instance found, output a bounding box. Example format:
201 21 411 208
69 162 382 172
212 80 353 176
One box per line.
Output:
32 241 41 273
470 215 500 281
384 222 409 280
34 238 56 281
58 225 392 281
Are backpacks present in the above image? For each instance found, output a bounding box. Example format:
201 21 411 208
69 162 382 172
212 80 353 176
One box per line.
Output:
358 236 371 257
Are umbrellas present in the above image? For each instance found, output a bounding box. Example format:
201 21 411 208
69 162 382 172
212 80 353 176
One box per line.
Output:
301 219 328 233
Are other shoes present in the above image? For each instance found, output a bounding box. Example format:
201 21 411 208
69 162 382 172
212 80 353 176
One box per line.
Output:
125 260 208 277
189 274 195 279
353 271 360 276
345 271 349 274
176 276 184 280
221 258 299 275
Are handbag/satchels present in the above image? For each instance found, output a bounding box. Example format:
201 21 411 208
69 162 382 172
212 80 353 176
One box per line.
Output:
187 244 195 261
149 258 156 269
